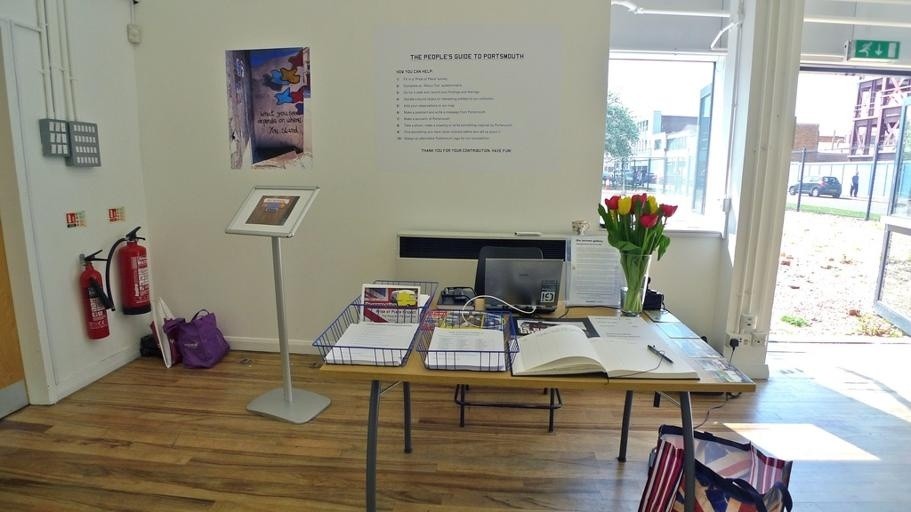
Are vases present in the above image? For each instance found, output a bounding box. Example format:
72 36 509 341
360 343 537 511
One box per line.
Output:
617 253 654 318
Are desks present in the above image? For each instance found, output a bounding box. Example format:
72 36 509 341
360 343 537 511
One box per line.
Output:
320 306 757 511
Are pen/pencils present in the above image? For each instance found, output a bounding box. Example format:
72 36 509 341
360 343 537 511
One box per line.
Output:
648 345 673 363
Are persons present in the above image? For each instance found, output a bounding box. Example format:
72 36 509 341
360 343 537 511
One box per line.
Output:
850 172 858 197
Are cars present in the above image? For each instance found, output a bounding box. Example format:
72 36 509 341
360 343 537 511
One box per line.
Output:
789 176 842 199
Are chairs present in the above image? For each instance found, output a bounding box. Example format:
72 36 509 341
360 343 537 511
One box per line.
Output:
454 244 564 432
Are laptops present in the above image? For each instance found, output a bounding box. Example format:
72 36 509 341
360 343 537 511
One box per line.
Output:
484 258 563 313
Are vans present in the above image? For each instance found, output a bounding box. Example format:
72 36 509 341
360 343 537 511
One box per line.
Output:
609 168 634 177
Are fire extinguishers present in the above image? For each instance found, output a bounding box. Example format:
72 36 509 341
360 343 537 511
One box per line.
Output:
106 226 151 315
79 249 114 339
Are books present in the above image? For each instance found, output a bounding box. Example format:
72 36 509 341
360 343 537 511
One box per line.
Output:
508 324 700 379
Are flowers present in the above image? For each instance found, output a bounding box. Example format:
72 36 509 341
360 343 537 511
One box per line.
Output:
598 193 679 312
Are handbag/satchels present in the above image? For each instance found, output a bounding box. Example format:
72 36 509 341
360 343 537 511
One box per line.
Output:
175 312 230 370
638 424 793 512
